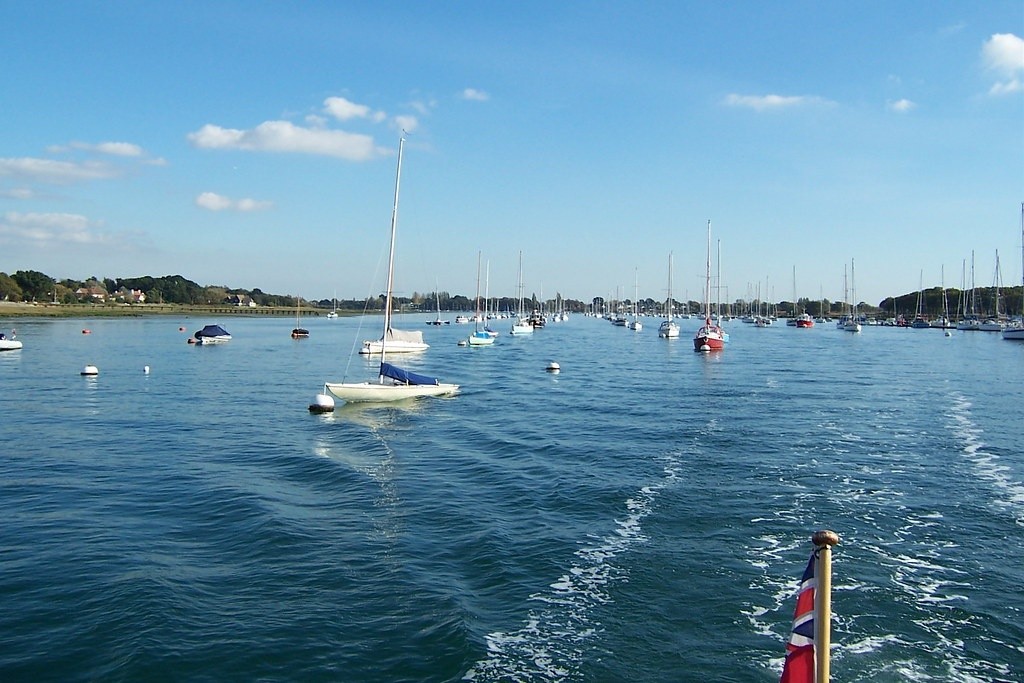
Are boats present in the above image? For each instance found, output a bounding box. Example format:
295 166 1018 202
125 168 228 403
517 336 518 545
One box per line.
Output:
0 339 23 350
357 231 429 353
325 135 461 402
194 324 233 343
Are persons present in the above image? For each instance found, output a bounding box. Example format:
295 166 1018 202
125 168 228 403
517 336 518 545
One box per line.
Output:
1007 320 1011 327
11 332 16 341
524 319 529 327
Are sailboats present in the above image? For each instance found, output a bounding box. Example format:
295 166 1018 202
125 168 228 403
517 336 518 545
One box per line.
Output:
326 290 339 318
290 293 309 337
423 200 1024 353
